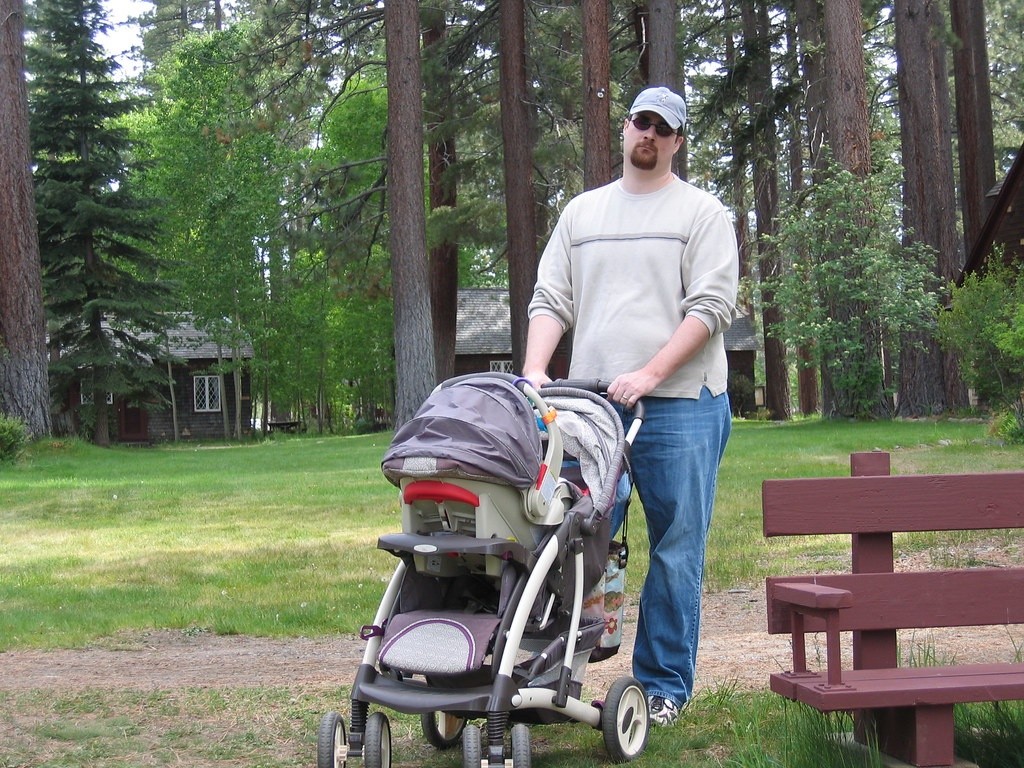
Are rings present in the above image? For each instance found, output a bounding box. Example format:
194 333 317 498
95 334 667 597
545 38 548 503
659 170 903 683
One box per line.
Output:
621 395 628 400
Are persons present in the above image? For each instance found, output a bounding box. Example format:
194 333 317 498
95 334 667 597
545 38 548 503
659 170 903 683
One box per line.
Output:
522 85 741 726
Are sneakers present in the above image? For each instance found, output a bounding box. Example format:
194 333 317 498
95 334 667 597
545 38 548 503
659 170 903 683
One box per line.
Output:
646 693 679 725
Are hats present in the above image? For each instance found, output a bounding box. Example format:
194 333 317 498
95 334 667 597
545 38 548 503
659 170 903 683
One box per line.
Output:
629 87 686 132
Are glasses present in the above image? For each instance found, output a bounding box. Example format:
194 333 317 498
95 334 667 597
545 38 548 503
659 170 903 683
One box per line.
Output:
629 117 681 137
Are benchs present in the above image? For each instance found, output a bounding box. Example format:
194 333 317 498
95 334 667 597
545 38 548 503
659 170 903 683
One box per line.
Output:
761 452 1024 767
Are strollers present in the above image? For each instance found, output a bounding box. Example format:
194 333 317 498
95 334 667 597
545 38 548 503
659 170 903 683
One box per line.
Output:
315 368 650 768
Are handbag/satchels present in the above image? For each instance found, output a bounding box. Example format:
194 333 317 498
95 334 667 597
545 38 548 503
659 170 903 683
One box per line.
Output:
581 500 631 664
